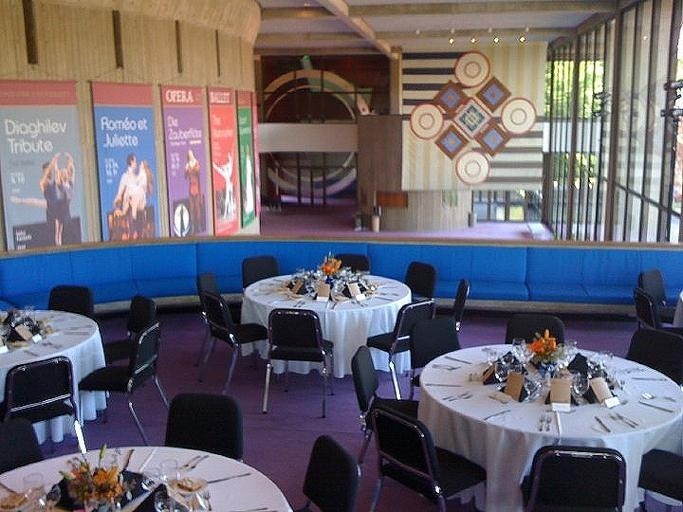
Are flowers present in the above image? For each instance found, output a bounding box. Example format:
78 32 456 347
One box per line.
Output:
527 331 561 369
317 254 342 279
66 443 136 512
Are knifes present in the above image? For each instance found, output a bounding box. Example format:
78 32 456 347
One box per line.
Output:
638 401 674 414
482 409 511 421
206 471 252 484
444 355 472 366
595 416 612 433
631 377 668 382
425 383 463 388
122 448 136 475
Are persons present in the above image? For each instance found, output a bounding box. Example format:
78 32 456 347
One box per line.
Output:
114 161 153 214
244 144 253 215
184 149 202 234
10 151 75 245
213 152 236 219
116 154 138 219
42 163 62 249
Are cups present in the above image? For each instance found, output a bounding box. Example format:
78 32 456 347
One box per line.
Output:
154 490 177 511
524 373 539 402
139 464 161 496
568 338 578 357
510 363 523 373
36 480 61 512
304 278 314 295
511 337 526 360
573 375 589 406
558 342 569 360
494 361 510 391
599 349 612 370
21 472 45 511
588 352 599 371
487 349 498 368
24 316 36 332
298 265 308 283
13 310 24 323
161 457 180 493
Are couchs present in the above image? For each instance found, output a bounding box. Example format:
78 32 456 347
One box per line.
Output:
0 234 683 322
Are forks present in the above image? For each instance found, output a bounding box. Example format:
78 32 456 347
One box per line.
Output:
186 454 210 472
537 412 545 432
448 394 475 402
546 414 553 431
433 364 461 369
442 391 470 400
181 456 201 471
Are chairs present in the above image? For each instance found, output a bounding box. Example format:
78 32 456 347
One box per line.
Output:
96 294 157 367
639 267 677 324
79 320 172 447
364 299 437 401
503 313 567 349
241 255 280 292
261 308 337 418
366 406 487 512
517 442 628 512
431 279 472 333
0 355 87 455
350 345 421 464
289 435 362 512
0 417 43 475
634 289 683 335
194 290 268 396
195 271 241 364
406 314 463 402
401 261 439 318
46 284 94 321
335 251 369 274
635 439 683 512
161 390 245 465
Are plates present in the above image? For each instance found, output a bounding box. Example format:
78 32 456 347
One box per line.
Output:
174 475 208 495
2 493 30 511
